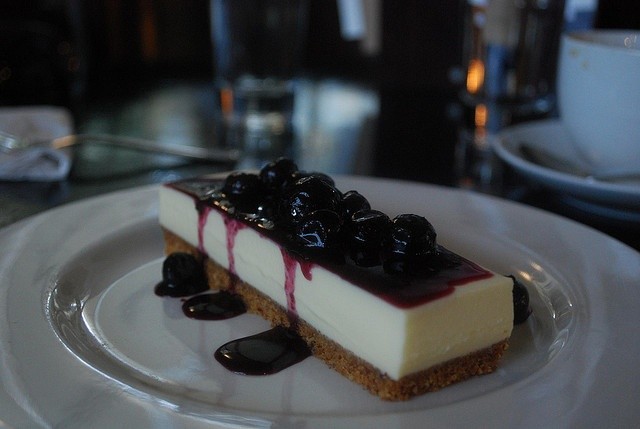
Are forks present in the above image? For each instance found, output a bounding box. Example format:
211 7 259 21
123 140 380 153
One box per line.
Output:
0 128 240 165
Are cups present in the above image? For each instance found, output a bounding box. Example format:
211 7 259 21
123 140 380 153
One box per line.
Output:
553 29 639 177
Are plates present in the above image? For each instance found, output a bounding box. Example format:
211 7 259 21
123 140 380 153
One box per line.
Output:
1 168 640 429
490 118 639 222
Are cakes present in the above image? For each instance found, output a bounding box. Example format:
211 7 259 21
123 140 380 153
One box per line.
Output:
152 155 532 403
